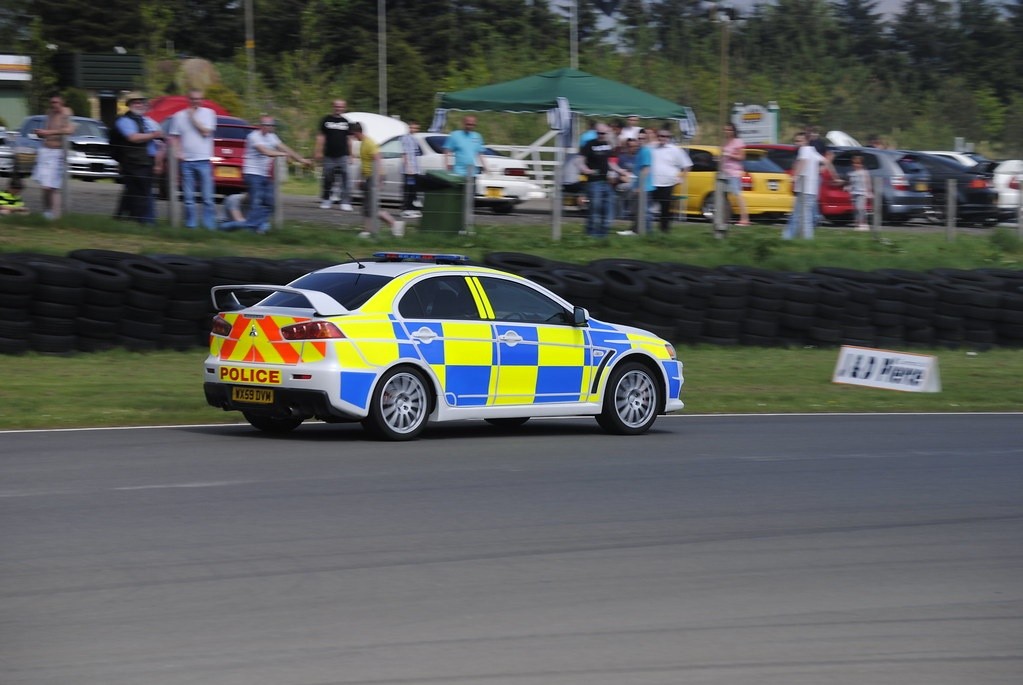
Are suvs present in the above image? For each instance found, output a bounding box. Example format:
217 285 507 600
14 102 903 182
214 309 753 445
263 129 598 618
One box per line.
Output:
833 147 934 225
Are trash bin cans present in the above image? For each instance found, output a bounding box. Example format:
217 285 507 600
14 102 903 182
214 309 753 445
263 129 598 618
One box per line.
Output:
419 168 467 239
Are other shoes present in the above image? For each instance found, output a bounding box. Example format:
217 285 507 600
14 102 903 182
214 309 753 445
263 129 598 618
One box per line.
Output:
852 223 871 232
617 230 638 236
404 211 422 218
339 203 352 213
392 220 406 238
319 199 333 209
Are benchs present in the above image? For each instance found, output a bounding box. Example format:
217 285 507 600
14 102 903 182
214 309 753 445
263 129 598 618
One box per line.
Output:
561 189 687 203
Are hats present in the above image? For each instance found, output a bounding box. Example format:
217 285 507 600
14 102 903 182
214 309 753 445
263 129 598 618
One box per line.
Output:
125 92 149 107
348 122 363 133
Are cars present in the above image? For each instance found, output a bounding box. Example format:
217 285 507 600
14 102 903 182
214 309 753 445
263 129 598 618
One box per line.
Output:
826 130 1023 226
147 96 277 199
203 251 685 439
743 145 875 225
320 112 529 213
0 115 126 186
668 144 798 222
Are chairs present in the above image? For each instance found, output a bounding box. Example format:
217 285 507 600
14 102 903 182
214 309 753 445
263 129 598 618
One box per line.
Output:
426 290 460 318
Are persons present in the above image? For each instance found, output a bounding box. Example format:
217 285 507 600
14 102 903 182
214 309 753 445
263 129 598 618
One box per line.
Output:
108 83 315 236
349 123 406 240
780 128 871 242
401 121 425 220
560 116 694 239
442 116 488 233
29 91 74 222
314 98 356 211
719 122 749 227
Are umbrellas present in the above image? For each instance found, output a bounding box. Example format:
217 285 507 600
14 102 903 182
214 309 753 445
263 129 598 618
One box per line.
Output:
435 65 697 240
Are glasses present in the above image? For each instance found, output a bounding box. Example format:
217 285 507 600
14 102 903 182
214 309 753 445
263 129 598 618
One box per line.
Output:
597 131 608 137
